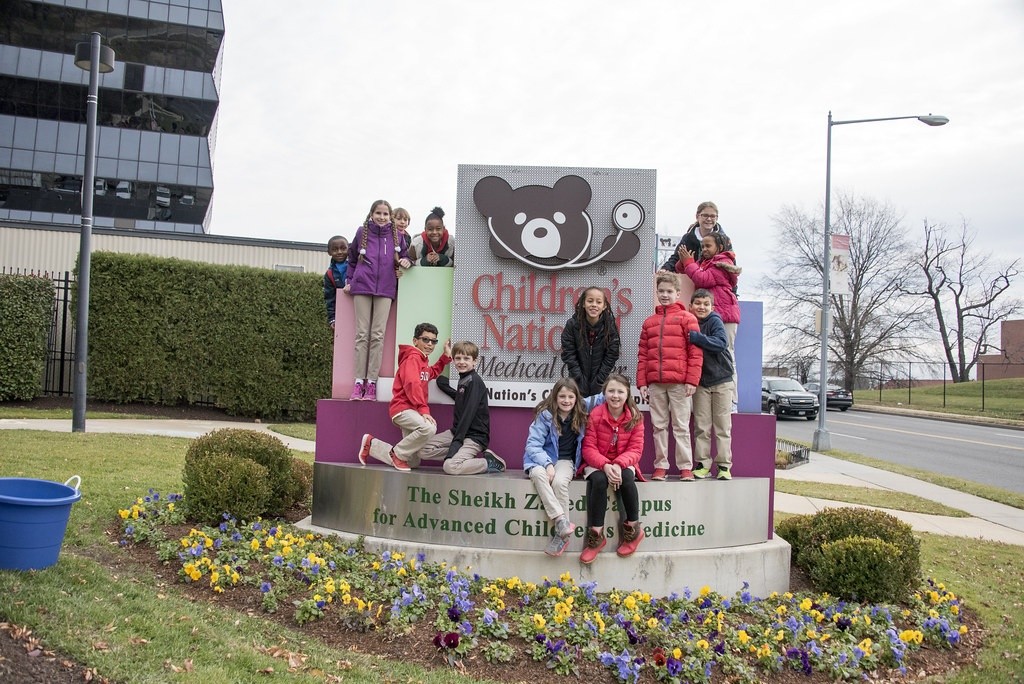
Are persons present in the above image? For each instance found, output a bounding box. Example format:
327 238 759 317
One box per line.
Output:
417 341 506 475
635 270 703 482
576 374 650 564
523 377 605 556
660 201 741 298
405 206 454 267
561 287 620 477
687 289 734 480
676 232 741 415
342 200 411 401
392 207 411 280
323 234 350 330
358 323 452 471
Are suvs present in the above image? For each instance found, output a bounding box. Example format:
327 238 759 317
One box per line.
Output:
761 375 820 421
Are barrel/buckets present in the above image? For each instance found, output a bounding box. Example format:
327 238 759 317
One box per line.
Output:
0 474 82 570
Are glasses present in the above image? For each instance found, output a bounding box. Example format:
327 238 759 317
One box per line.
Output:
416 337 438 345
698 214 717 218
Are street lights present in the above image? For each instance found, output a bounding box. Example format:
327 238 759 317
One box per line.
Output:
812 110 951 452
71 31 116 431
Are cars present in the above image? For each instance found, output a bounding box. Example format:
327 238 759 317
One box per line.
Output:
803 383 854 411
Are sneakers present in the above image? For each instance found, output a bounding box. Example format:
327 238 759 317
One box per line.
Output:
483 449 506 472
679 469 695 481
362 382 377 402
556 514 575 540
716 465 732 480
350 382 365 401
692 463 712 478
651 468 668 480
358 434 374 465
731 402 738 414
545 534 570 557
388 449 412 471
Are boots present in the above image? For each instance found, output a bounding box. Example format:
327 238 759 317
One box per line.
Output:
617 519 644 557
580 527 606 563
